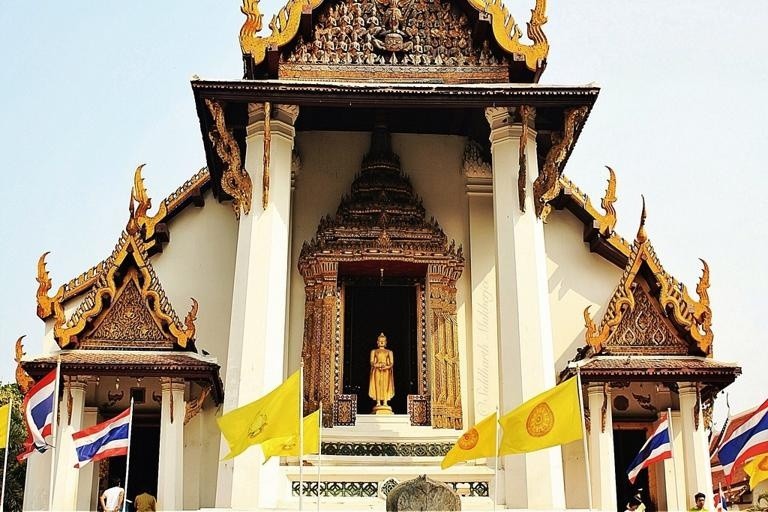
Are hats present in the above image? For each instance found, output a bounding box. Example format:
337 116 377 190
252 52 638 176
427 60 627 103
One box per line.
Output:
634 493 642 503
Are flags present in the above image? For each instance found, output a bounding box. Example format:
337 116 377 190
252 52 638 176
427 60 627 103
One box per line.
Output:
716 396 768 489
70 403 131 470
14 366 58 465
496 374 585 458
624 407 674 487
439 406 497 471
713 481 729 512
0 401 11 450
258 405 322 466
742 450 768 492
215 365 303 463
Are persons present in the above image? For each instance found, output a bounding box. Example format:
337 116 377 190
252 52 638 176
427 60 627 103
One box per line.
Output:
688 491 710 512
98 476 126 512
133 482 157 511
627 493 646 512
625 499 639 512
369 334 397 406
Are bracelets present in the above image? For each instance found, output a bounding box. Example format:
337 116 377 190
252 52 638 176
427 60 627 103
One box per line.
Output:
116 505 121 509
